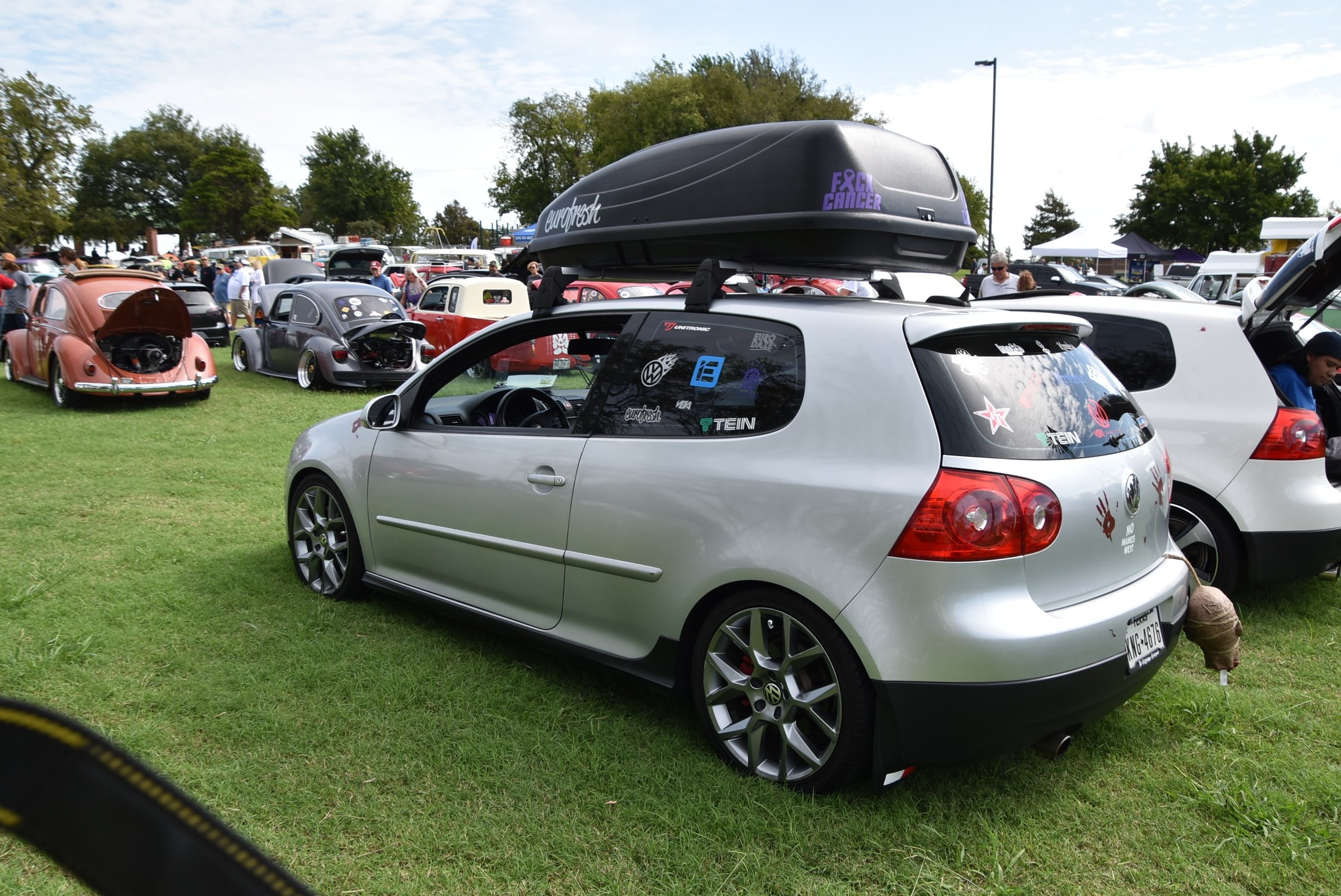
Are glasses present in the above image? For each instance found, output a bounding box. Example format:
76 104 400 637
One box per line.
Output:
216 268 221 270
530 267 536 269
57 255 63 258
201 259 207 261
991 264 1006 271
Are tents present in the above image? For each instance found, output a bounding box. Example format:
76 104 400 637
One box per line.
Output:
514 223 537 242
1096 231 1178 284
1164 246 1206 262
1032 226 1128 282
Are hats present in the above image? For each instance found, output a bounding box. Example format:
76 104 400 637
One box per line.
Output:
0 253 15 262
215 264 225 269
488 260 498 268
233 257 241 262
1287 332 1341 360
369 261 381 269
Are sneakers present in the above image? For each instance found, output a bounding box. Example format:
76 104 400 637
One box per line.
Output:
244 324 250 328
228 327 237 332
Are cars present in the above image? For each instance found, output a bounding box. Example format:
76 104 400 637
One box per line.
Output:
284 118 1189 797
969 214 1341 585
0 226 972 364
965 250 1330 307
230 281 427 393
405 277 591 380
3 267 221 411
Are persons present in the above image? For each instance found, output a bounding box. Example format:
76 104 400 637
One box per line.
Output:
1016 270 1037 291
463 257 475 270
970 256 978 274
402 266 434 308
838 280 875 299
487 261 506 278
979 252 1021 298
0 273 18 340
474 260 483 269
527 262 544 311
978 263 987 275
1041 258 1091 276
0 252 38 335
59 247 84 274
370 261 395 296
1269 331 1341 460
168 255 266 330
757 273 763 284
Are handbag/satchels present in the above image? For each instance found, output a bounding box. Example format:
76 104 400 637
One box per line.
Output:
408 294 422 306
